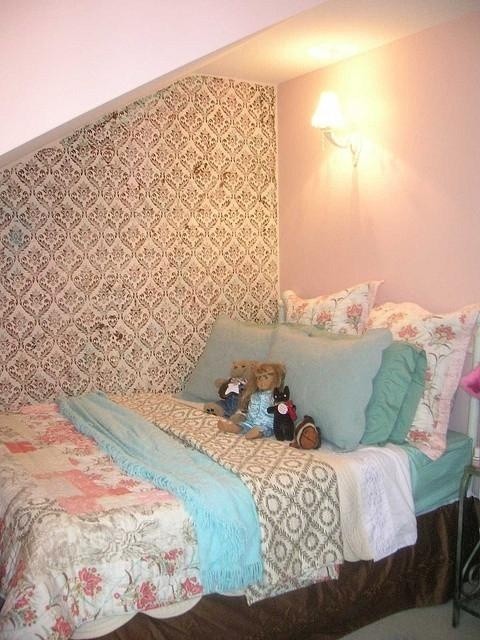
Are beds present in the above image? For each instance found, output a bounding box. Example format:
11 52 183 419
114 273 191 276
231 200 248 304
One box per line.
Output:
0 300 480 639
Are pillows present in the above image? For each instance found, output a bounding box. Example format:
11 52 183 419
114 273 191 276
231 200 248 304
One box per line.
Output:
283 279 388 334
174 315 314 405
268 324 391 453
309 327 427 445
361 300 480 462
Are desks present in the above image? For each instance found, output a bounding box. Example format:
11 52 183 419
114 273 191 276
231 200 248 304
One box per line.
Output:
451 463 480 628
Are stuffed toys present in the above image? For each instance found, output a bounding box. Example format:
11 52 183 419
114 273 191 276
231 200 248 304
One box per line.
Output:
266 385 297 441
288 414 323 449
203 357 261 423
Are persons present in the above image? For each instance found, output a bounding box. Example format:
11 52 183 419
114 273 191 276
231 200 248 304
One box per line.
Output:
216 361 287 440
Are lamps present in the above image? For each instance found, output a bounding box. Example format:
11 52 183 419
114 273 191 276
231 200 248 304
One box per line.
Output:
312 91 362 159
